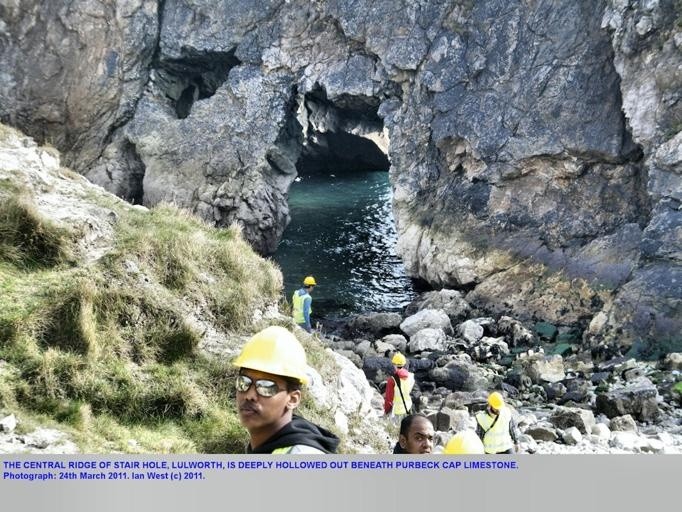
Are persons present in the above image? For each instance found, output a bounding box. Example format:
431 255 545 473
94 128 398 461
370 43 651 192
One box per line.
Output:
391 414 436 453
292 275 317 332
232 326 340 454
474 390 523 454
381 351 422 417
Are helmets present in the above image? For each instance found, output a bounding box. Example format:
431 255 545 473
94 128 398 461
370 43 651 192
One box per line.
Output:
233 326 308 384
488 392 504 410
445 432 484 454
304 277 317 285
391 353 406 365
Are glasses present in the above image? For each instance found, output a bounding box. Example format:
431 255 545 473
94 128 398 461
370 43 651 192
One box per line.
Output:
235 375 288 397
